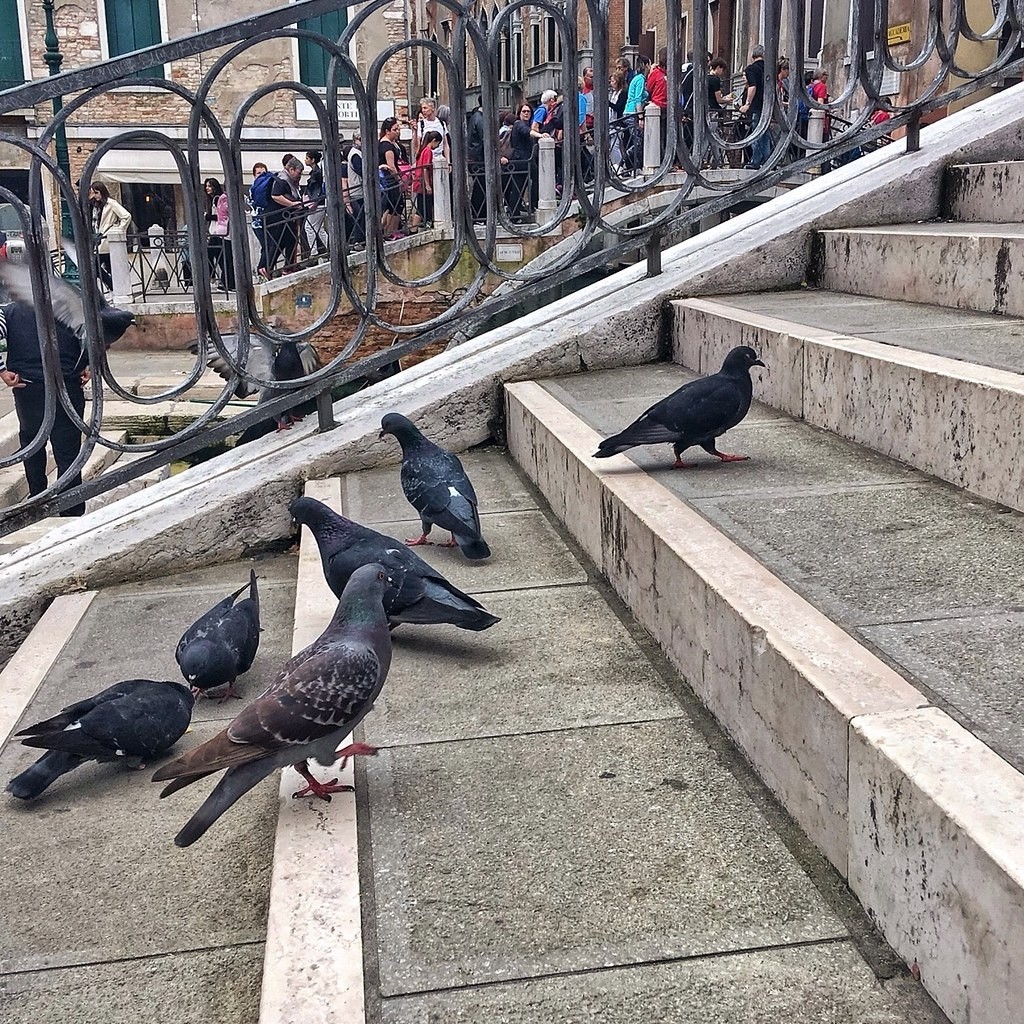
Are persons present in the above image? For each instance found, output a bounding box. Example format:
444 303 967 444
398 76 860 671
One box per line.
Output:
286 44 899 266
179 224 195 287
217 179 238 295
251 161 273 281
87 180 132 301
0 301 92 516
203 176 226 282
260 158 306 282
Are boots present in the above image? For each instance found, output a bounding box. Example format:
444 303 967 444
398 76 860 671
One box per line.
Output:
384 214 404 239
408 214 421 234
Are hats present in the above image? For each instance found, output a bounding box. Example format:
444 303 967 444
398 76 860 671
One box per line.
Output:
287 157 304 170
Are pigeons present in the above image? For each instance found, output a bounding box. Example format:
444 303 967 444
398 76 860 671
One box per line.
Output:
590 347 768 469
174 569 261 703
377 411 491 560
152 564 392 848
1 263 134 369
287 497 505 632
8 679 193 801
186 331 317 432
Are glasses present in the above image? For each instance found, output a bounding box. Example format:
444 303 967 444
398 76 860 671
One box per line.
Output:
295 170 303 175
522 110 530 113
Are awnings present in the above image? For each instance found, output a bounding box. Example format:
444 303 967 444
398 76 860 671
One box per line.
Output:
94 148 347 187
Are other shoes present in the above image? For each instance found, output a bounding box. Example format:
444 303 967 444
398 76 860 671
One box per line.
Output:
282 270 293 276
555 183 578 200
258 267 271 281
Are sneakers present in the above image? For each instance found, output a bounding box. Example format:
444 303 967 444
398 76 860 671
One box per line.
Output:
217 283 236 293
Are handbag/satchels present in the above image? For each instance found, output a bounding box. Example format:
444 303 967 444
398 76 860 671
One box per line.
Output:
397 164 411 192
209 221 229 237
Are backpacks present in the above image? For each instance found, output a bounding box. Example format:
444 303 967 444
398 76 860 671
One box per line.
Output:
552 104 563 130
499 131 514 159
251 171 284 208
860 111 883 151
798 81 820 120
